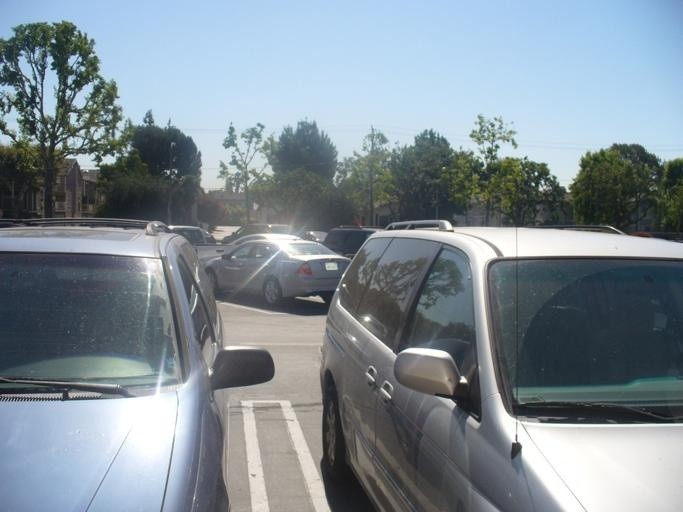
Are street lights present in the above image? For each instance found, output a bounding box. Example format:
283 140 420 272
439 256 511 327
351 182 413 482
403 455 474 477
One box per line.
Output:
166 141 176 224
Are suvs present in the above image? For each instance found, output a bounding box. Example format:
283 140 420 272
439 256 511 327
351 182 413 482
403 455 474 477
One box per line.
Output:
321 224 385 264
316 215 682 510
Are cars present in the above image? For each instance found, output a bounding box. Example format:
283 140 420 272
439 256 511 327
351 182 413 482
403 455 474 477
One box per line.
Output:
0 216 274 511
166 223 329 243
203 240 352 306
194 232 303 266
